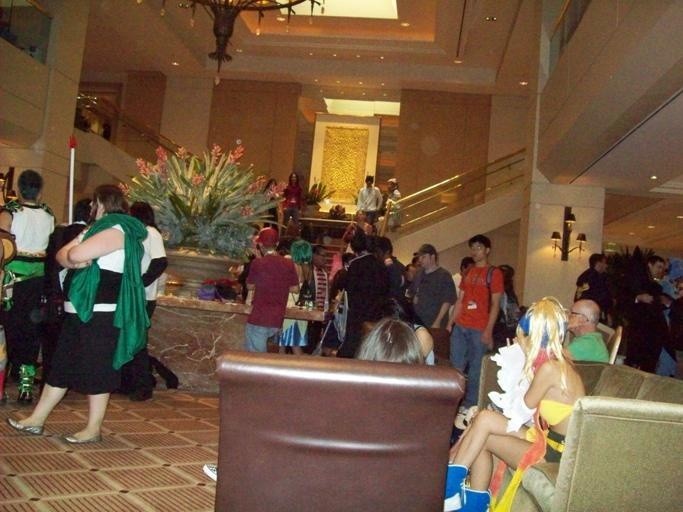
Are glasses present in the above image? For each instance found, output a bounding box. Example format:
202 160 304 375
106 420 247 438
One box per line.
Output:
470 246 485 251
567 310 590 322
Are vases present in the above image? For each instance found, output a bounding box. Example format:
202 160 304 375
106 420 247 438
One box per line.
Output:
301 205 321 217
162 249 241 288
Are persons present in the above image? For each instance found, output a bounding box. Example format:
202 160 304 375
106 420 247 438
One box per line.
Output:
367 298 436 364
306 245 328 355
443 296 585 512
669 278 682 379
447 235 503 408
411 254 420 276
343 211 373 242
326 253 355 357
382 178 400 234
564 299 609 362
116 201 168 402
43 199 91 386
265 180 279 231
246 230 300 352
355 175 382 225
339 234 388 360
492 264 522 352
362 319 427 366
411 244 454 328
369 240 407 317
404 264 414 287
573 255 616 325
279 239 313 354
0 169 55 405
451 257 473 297
280 173 305 236
622 258 665 373
6 185 132 445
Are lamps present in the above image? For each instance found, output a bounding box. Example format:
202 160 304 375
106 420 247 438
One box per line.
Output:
135 0 328 86
550 214 586 261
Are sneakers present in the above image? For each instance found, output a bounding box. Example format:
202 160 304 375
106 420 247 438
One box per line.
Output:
444 465 468 511
204 463 218 482
449 491 490 512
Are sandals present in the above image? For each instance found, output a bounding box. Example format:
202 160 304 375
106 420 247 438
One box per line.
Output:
8 417 44 435
64 433 103 444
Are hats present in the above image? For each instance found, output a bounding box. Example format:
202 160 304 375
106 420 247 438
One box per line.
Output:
413 244 437 256
255 227 280 247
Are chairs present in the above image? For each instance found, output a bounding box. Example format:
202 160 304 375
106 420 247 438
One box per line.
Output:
605 326 622 365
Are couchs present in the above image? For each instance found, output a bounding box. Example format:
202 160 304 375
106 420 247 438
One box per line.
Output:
210 355 467 512
477 354 682 511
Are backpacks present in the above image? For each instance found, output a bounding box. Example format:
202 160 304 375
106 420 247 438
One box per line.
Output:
496 287 520 335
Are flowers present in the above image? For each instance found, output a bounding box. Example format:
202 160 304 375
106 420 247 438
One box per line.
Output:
329 204 346 238
117 144 289 260
301 182 336 205
